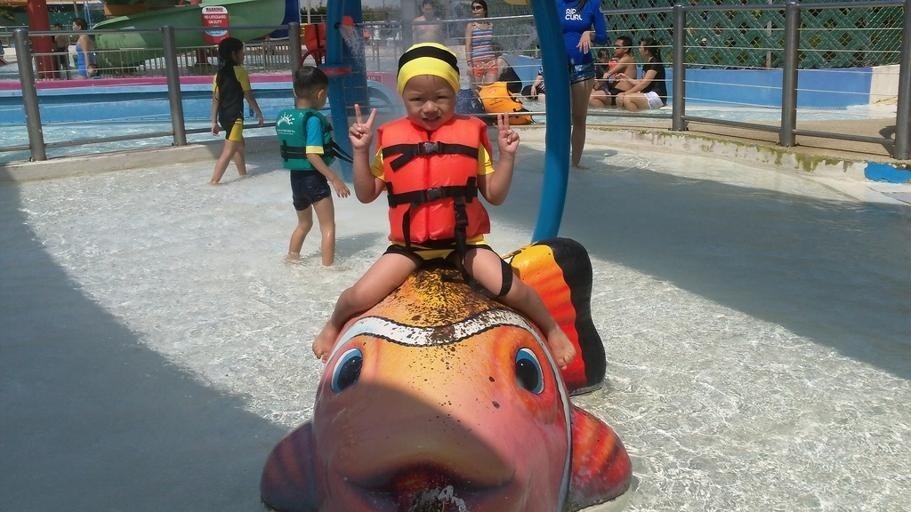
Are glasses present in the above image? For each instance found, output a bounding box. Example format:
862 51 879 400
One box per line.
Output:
472 6 483 11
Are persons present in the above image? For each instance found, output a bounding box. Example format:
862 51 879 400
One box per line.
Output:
465 0 498 89
275 66 351 267
596 47 613 95
312 43 577 369
411 0 443 44
54 22 71 80
209 38 265 184
87 64 101 79
588 35 636 108
615 37 667 111
492 42 522 93
73 18 97 79
521 61 545 104
555 0 606 171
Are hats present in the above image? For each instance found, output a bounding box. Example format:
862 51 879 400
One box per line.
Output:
397 43 460 95
